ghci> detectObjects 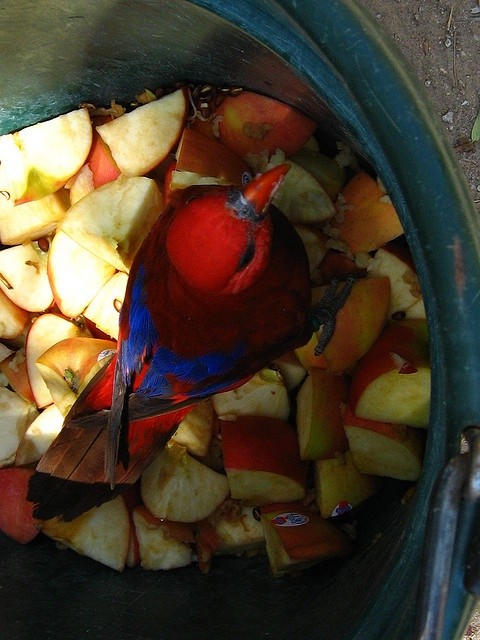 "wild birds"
[27,163,357,524]
